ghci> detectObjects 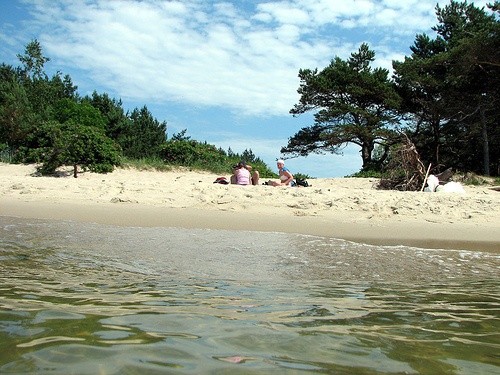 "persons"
[244,163,260,186]
[264,160,294,188]
[230,161,251,185]
